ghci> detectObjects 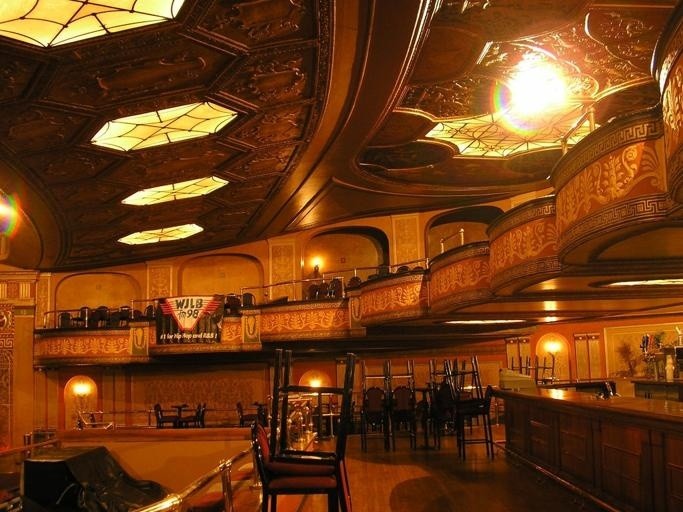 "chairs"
[154,402,206,429]
[309,266,424,299]
[361,355,497,461]
[58,293,256,328]
[512,355,554,386]
[236,349,357,512]
